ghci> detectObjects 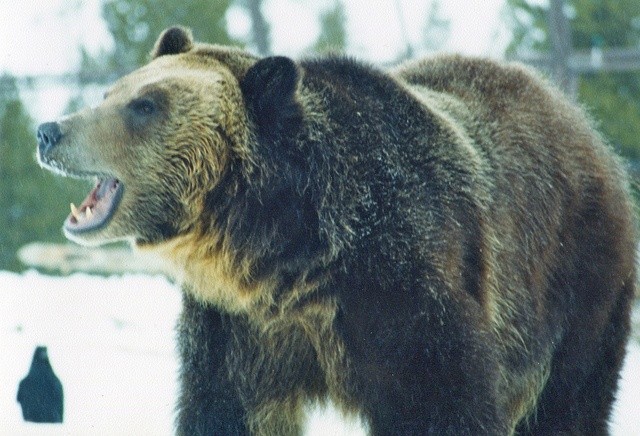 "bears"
[35,22,636,436]
